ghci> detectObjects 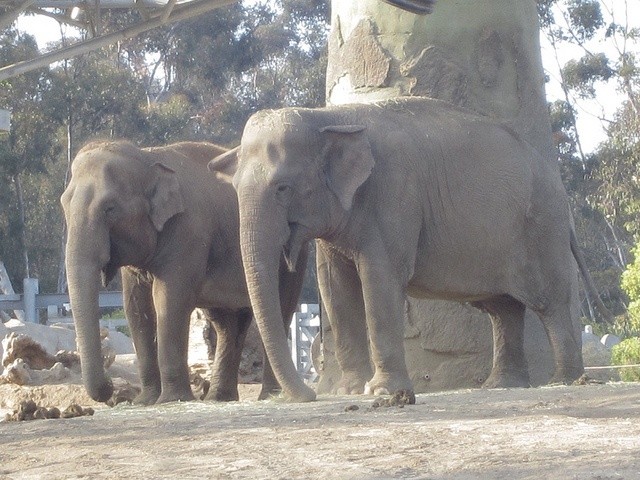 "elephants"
[59,137,312,407]
[206,93,616,403]
